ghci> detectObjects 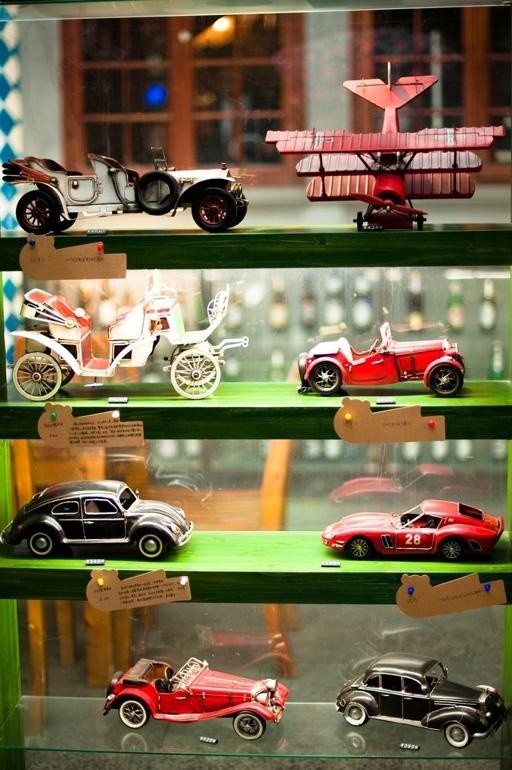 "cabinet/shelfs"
[0,0,512,770]
